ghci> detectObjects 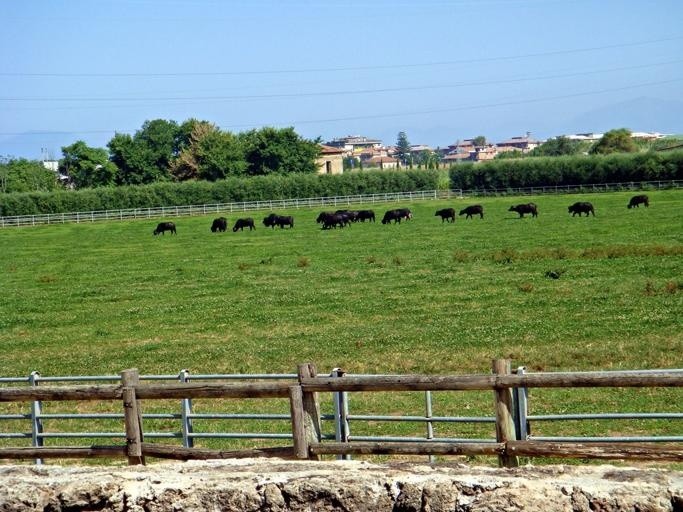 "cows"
[458,204,485,220]
[434,208,457,223]
[232,217,257,231]
[315,207,376,230]
[210,216,228,232]
[508,202,539,219]
[153,221,176,237]
[381,207,412,225]
[626,193,650,209]
[262,213,294,229]
[568,201,597,218]
